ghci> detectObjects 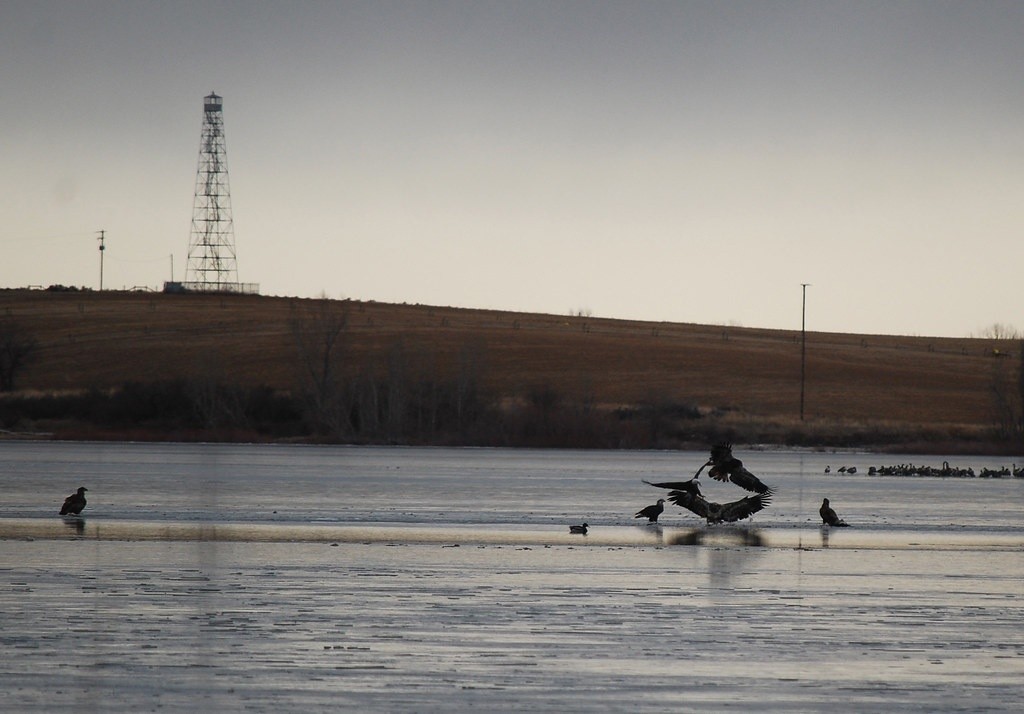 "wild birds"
[59,487,88,515]
[819,498,851,528]
[569,523,590,535]
[824,460,1024,478]
[634,441,777,526]
